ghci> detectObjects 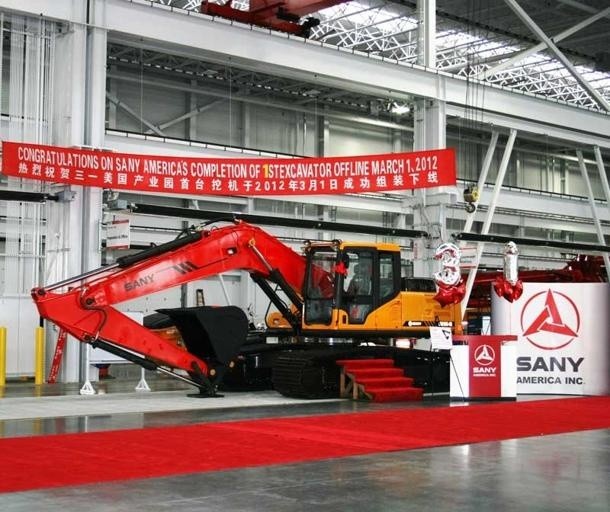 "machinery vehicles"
[29,210,468,398]
